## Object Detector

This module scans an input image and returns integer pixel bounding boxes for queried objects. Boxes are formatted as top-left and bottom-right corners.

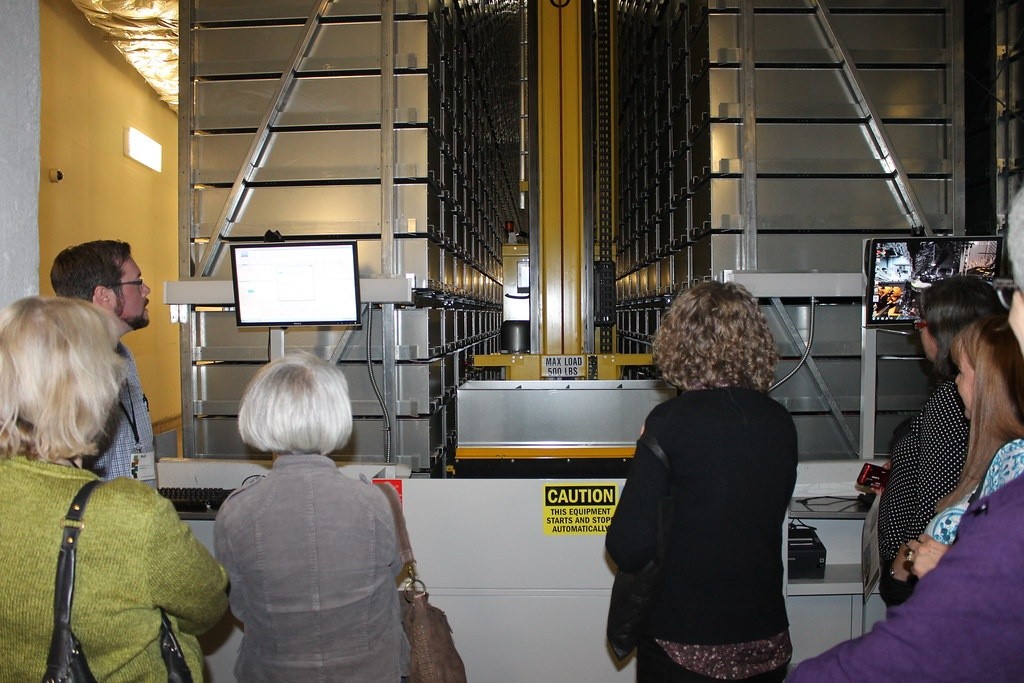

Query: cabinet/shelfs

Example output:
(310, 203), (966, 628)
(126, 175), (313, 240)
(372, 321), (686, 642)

(786, 594), (888, 664)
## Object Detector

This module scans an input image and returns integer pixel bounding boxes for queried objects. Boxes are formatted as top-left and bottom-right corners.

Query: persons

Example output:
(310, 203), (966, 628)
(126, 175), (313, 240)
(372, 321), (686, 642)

(0, 240), (230, 683)
(605, 280), (799, 682)
(212, 353), (412, 683)
(782, 186), (1023, 683)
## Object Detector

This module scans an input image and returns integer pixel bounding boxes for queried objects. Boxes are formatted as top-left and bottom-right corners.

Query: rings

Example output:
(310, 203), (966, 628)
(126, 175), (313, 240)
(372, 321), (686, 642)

(907, 551), (912, 560)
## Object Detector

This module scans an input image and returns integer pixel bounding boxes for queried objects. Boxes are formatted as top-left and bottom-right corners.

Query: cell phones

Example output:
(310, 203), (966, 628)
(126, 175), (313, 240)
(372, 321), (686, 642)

(857, 463), (891, 490)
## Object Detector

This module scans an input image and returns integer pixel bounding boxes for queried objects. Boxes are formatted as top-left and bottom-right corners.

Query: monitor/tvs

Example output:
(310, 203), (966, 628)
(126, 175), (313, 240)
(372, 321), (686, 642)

(866, 234), (1004, 327)
(230, 240), (362, 328)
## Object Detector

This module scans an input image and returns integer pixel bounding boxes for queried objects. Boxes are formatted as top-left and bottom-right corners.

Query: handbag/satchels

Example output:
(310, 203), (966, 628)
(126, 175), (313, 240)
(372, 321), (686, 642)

(41, 480), (194, 683)
(377, 481), (467, 683)
(606, 432), (672, 662)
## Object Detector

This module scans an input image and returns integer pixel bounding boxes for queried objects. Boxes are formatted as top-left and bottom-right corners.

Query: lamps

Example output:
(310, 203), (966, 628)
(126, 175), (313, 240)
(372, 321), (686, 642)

(123, 126), (163, 173)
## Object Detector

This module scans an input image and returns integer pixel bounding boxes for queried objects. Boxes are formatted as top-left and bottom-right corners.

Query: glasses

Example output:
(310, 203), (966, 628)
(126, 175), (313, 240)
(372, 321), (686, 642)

(993, 278), (1024, 309)
(915, 320), (930, 332)
(110, 278), (144, 292)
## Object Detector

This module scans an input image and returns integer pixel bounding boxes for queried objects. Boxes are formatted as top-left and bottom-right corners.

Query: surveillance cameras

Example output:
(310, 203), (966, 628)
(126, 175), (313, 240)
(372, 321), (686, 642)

(49, 168), (64, 182)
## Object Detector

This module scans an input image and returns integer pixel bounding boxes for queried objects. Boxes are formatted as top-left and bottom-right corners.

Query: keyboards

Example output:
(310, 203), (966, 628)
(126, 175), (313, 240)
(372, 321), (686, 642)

(159, 486), (234, 511)
